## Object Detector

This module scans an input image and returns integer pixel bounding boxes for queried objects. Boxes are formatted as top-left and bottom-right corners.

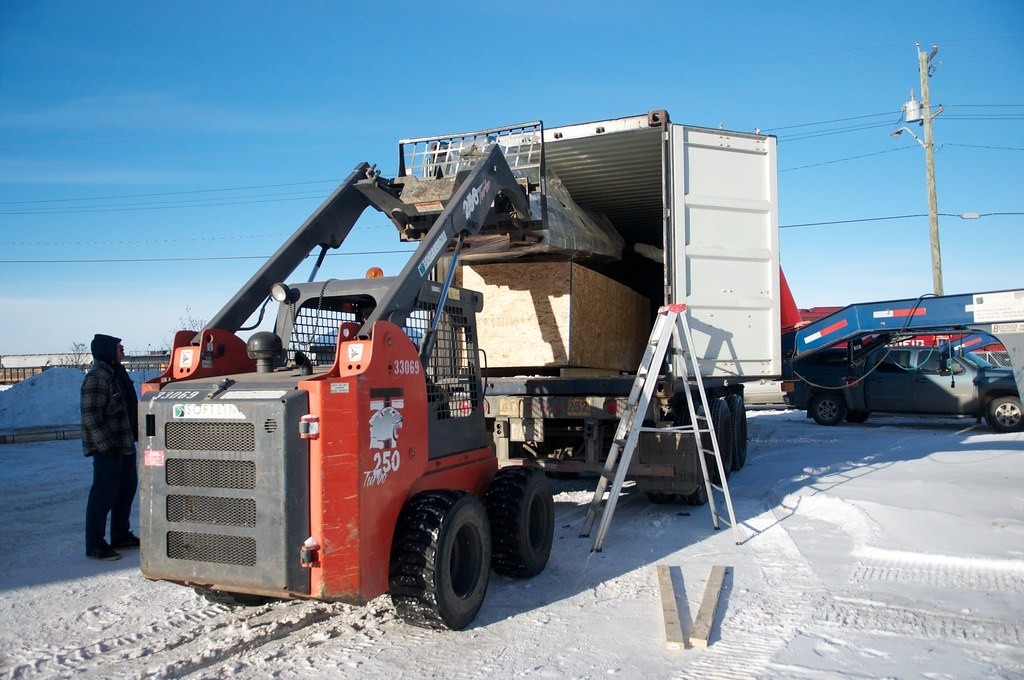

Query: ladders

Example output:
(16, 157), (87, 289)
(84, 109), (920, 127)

(577, 304), (740, 552)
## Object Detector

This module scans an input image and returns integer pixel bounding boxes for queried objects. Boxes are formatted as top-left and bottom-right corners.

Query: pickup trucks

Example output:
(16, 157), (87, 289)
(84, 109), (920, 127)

(780, 346), (1024, 433)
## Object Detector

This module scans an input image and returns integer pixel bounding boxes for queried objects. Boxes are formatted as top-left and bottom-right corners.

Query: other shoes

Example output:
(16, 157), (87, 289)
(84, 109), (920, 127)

(110, 530), (140, 549)
(86, 546), (121, 561)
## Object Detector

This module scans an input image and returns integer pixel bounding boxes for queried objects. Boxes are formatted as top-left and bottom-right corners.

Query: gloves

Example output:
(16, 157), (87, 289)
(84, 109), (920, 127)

(104, 445), (121, 459)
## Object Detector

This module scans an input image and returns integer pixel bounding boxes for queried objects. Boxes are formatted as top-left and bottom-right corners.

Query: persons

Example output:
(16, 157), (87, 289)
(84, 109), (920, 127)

(82, 334), (140, 561)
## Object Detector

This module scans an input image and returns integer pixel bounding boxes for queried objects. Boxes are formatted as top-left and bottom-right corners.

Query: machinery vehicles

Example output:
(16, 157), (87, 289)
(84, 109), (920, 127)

(138, 104), (782, 631)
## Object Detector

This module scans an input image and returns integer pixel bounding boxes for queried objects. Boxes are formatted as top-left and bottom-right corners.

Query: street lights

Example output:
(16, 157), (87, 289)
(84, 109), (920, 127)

(892, 126), (943, 298)
